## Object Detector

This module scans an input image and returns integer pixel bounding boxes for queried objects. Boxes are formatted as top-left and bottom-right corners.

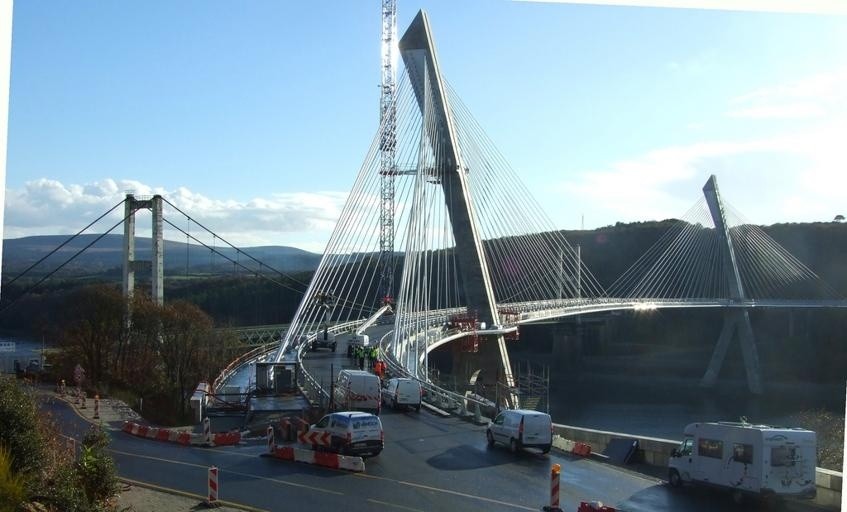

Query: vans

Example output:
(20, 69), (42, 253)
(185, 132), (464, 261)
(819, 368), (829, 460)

(334, 369), (422, 411)
(668, 422), (816, 505)
(486, 409), (553, 454)
(311, 411), (384, 455)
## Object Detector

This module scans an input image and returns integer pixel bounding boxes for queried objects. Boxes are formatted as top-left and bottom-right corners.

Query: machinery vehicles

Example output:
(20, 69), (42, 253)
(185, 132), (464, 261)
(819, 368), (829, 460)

(312, 293), (336, 352)
(348, 304), (393, 346)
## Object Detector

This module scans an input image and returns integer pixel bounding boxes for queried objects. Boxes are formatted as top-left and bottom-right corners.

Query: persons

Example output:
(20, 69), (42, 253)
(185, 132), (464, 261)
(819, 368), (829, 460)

(379, 378), (387, 389)
(348, 342), (386, 377)
